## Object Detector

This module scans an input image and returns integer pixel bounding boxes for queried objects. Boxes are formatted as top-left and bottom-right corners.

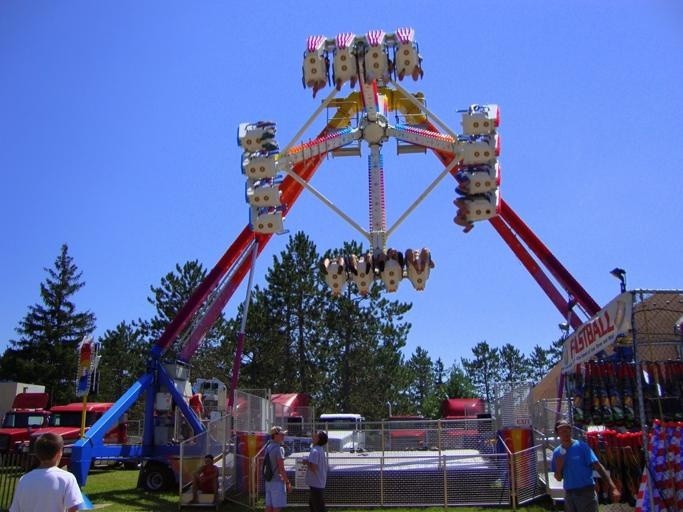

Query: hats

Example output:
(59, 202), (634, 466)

(205, 455), (214, 458)
(555, 420), (571, 429)
(271, 426), (288, 434)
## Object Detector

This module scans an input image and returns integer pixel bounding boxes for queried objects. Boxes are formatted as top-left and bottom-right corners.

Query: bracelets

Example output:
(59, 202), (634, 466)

(285, 480), (290, 485)
(307, 461), (311, 465)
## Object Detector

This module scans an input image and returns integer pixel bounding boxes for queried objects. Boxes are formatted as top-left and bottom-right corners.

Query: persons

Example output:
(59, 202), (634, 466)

(264, 425), (294, 511)
(9, 432), (84, 511)
(189, 392), (208, 444)
(551, 424), (624, 510)
(259, 41), (474, 276)
(300, 431), (327, 510)
(187, 453), (222, 505)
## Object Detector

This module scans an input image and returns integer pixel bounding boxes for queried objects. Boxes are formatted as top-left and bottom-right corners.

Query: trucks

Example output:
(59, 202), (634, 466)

(0, 391), (145, 473)
(269, 392), (492, 453)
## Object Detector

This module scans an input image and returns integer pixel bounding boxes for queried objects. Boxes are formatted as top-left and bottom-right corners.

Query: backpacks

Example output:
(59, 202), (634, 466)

(263, 446), (278, 481)
